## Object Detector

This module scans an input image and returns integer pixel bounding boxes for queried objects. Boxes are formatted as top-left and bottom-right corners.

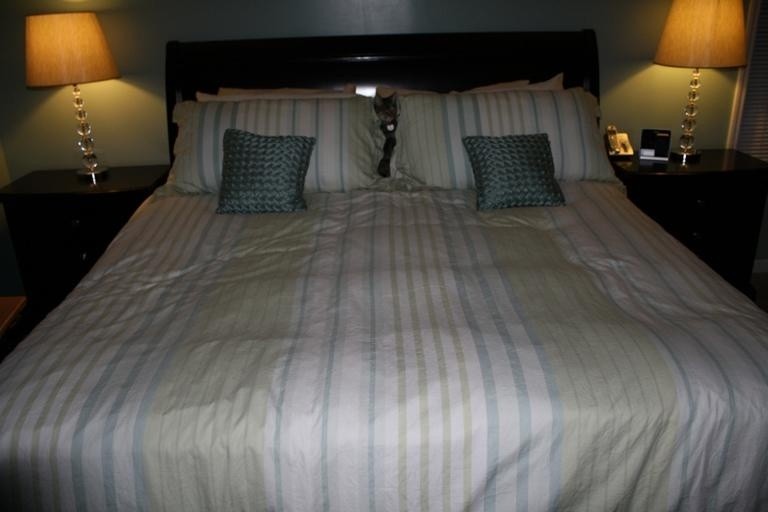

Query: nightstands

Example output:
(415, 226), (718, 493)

(608, 146), (767, 294)
(1, 166), (171, 315)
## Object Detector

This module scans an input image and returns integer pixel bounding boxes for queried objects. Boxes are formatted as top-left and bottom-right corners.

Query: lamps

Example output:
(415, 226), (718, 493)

(654, 2), (751, 164)
(23, 13), (120, 186)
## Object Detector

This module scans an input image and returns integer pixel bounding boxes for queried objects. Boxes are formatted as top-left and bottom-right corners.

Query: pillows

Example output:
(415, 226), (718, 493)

(387, 92), (617, 191)
(173, 96), (386, 196)
(462, 132), (567, 214)
(214, 128), (317, 214)
(500, 74), (568, 92)
(195, 92), (361, 102)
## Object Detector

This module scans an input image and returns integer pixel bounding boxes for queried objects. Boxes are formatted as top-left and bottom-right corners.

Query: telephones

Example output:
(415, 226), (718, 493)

(606, 120), (634, 156)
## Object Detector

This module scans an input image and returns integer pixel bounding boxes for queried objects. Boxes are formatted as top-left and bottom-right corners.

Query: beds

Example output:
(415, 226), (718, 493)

(2, 30), (767, 511)
